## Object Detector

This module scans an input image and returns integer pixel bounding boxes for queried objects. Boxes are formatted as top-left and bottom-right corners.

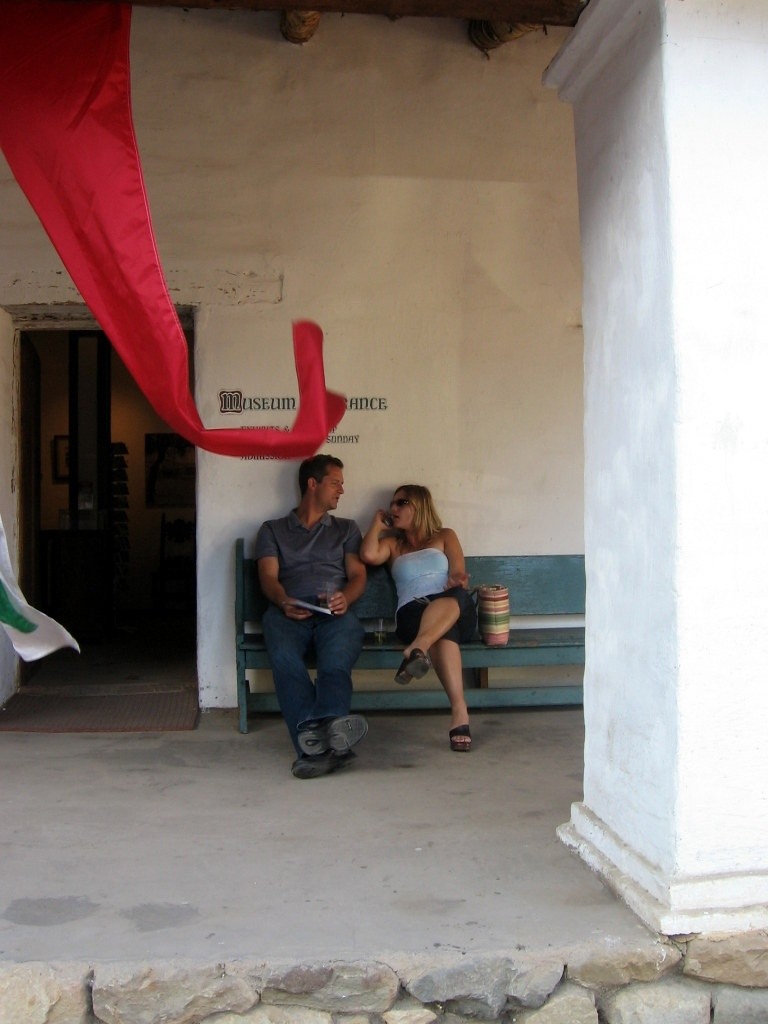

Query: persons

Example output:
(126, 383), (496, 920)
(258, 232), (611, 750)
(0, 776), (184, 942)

(360, 484), (478, 752)
(254, 455), (369, 780)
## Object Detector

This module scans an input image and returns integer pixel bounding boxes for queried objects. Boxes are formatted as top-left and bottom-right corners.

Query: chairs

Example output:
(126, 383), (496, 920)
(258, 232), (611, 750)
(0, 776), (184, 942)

(149, 513), (196, 617)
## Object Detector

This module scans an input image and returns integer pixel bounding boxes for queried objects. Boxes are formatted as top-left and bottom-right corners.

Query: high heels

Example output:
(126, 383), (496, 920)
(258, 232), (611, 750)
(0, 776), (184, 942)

(394, 648), (431, 685)
(449, 724), (472, 750)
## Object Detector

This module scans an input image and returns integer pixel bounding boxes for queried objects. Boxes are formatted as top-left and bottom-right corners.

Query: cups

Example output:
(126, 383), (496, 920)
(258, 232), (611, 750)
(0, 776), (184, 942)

(373, 618), (387, 647)
(320, 582), (337, 609)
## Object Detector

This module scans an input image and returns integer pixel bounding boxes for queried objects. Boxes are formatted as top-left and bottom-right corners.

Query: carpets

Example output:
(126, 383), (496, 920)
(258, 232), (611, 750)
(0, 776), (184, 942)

(0, 688), (200, 733)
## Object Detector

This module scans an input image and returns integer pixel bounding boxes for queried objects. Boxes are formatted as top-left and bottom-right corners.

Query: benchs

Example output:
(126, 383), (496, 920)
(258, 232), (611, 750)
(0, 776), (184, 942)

(233, 538), (586, 736)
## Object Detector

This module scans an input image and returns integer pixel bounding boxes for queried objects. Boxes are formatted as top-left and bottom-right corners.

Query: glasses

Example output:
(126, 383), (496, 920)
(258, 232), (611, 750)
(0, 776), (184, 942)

(390, 499), (411, 507)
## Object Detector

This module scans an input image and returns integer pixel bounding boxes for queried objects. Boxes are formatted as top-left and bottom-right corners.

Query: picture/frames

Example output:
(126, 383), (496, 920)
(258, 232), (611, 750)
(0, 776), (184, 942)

(51, 433), (69, 485)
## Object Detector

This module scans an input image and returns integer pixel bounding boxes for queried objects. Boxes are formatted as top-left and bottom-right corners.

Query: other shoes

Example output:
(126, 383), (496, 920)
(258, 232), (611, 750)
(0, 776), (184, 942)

(297, 713), (367, 757)
(292, 746), (359, 780)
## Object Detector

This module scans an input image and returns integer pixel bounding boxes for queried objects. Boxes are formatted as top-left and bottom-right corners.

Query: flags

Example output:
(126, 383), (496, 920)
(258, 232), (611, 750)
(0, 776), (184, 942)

(0, 515), (82, 662)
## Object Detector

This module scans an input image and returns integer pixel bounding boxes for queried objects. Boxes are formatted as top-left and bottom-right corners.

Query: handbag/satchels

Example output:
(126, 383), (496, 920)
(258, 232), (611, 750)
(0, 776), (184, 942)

(470, 585), (509, 646)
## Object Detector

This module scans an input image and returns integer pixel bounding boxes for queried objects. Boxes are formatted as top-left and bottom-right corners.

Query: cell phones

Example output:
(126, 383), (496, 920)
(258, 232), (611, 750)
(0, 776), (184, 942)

(385, 518), (393, 526)
